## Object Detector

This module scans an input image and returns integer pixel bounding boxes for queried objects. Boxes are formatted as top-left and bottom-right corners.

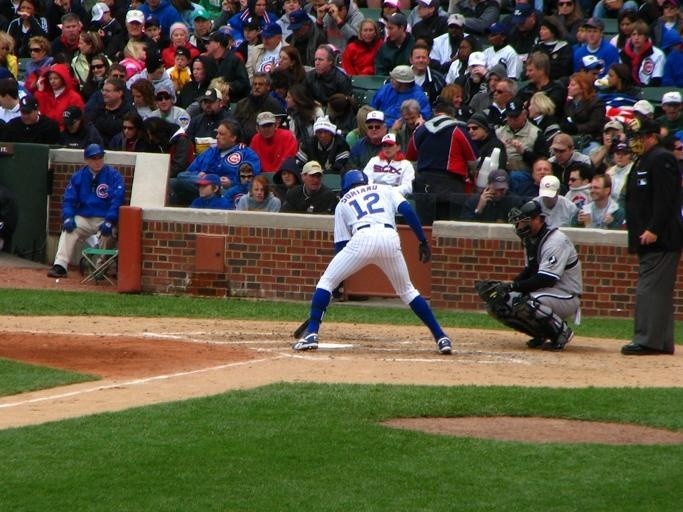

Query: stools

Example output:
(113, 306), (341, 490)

(79, 247), (118, 286)
(77, 239), (101, 277)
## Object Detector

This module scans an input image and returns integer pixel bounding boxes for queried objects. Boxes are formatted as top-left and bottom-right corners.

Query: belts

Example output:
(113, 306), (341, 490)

(357, 222), (396, 231)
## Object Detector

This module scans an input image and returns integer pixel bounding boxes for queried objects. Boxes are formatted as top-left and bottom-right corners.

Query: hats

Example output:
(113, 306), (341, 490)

(258, 21), (282, 38)
(468, 51), (487, 67)
(541, 15), (564, 40)
(504, 98), (525, 118)
(147, 55), (164, 74)
(602, 120), (624, 132)
(384, 0), (401, 9)
(90, 2), (111, 22)
(614, 142), (628, 154)
(219, 26), (236, 43)
(144, 14), (160, 24)
(538, 175), (561, 199)
(193, 8), (210, 23)
(84, 143), (106, 158)
(364, 109), (386, 124)
(391, 65), (416, 84)
(660, 91), (683, 105)
(446, 12), (466, 27)
(313, 115), (338, 135)
(416, 0), (439, 10)
(549, 133), (574, 151)
(487, 169), (510, 190)
(242, 13), (262, 30)
(62, 105), (83, 126)
(379, 132), (403, 146)
(575, 55), (605, 71)
(192, 173), (221, 186)
(511, 3), (533, 24)
(631, 100), (655, 115)
(467, 109), (490, 132)
(201, 30), (230, 49)
(199, 88), (223, 103)
(485, 22), (507, 35)
(286, 10), (310, 29)
(256, 111), (276, 126)
(174, 46), (191, 62)
(301, 160), (324, 176)
(583, 18), (606, 32)
(154, 85), (172, 97)
(384, 12), (407, 31)
(169, 22), (190, 43)
(125, 9), (145, 24)
(15, 95), (39, 114)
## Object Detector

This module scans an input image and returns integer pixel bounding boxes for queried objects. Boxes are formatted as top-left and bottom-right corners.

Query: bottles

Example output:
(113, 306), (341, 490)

(306, 205), (315, 213)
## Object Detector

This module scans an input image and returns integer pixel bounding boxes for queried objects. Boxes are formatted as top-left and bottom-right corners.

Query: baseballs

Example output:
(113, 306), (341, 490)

(55, 278), (60, 283)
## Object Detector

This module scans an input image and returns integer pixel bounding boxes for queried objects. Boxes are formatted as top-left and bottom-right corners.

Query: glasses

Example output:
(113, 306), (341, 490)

(157, 95), (170, 102)
(559, 1), (574, 6)
(113, 74), (125, 79)
(570, 177), (582, 183)
(240, 174), (253, 179)
(30, 48), (41, 53)
(123, 125), (134, 130)
(91, 64), (104, 69)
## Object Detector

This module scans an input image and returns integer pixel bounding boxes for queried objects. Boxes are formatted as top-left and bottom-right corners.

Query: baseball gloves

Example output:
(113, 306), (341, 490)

(477, 280), (516, 303)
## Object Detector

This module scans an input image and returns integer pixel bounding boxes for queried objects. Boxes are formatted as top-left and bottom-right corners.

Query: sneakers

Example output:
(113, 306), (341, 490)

(291, 329), (320, 351)
(437, 336), (453, 354)
(543, 324), (575, 352)
(621, 337), (675, 357)
(527, 318), (555, 349)
(47, 264), (68, 279)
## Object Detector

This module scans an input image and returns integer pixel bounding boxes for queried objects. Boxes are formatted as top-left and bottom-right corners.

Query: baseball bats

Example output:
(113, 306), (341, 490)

(293, 287), (344, 339)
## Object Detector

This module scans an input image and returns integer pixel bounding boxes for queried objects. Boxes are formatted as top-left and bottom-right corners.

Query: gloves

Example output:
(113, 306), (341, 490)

(97, 220), (114, 238)
(62, 217), (78, 234)
(417, 240), (431, 264)
(331, 280), (344, 299)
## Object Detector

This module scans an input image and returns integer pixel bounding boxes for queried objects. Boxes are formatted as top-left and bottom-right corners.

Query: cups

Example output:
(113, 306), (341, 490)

(476, 147), (500, 187)
(583, 204), (593, 226)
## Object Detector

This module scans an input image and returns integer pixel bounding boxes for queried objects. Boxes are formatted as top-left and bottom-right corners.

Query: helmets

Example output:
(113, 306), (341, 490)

(507, 200), (543, 239)
(340, 169), (368, 193)
